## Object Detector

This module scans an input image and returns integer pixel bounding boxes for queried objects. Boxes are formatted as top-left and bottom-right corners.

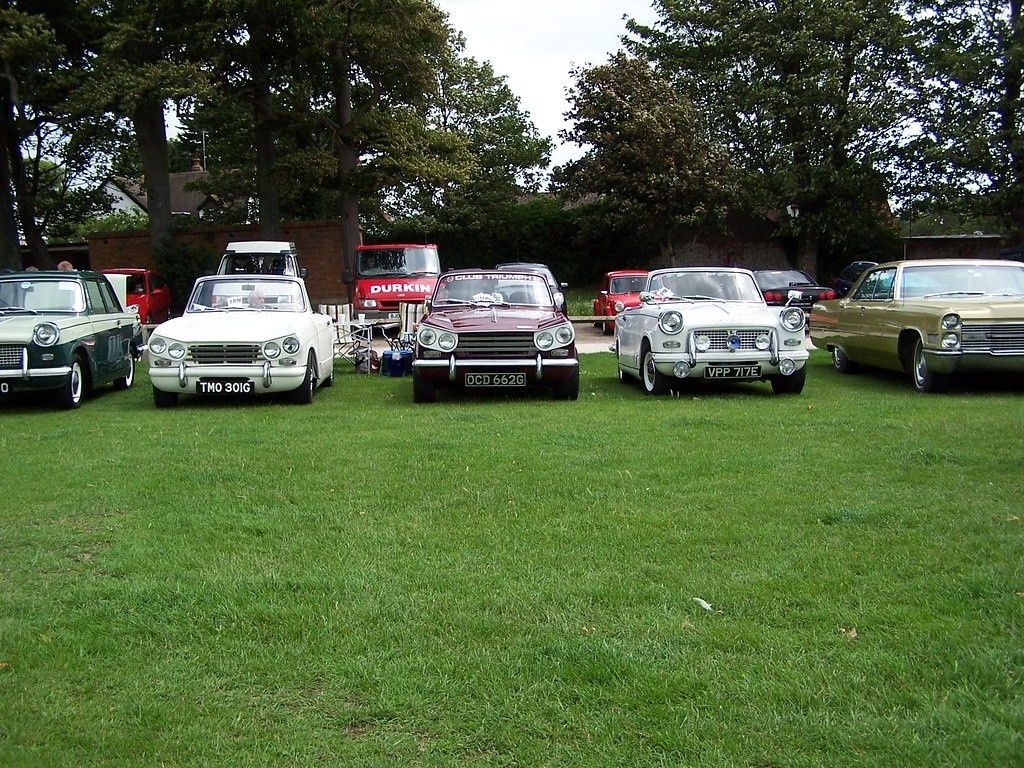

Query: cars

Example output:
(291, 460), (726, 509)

(809, 258), (1024, 392)
(148, 275), (334, 408)
(412, 268), (580, 403)
(593, 271), (670, 336)
(753, 270), (836, 328)
(613, 266), (809, 396)
(487, 263), (568, 320)
(100, 268), (171, 332)
(0, 269), (145, 409)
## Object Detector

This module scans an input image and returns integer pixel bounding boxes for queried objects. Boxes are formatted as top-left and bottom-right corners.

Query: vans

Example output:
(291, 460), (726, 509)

(211, 241), (307, 310)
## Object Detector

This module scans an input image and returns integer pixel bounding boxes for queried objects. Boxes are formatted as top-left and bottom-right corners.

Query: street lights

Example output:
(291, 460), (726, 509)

(201, 127), (209, 171)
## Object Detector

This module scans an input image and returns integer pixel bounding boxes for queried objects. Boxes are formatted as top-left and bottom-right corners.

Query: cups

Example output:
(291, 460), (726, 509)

(393, 353), (402, 360)
(338, 314), (345, 324)
(358, 314), (365, 322)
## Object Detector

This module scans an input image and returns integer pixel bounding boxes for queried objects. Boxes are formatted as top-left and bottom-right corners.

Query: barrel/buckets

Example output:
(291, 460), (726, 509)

(390, 358), (403, 377)
(383, 350), (395, 375)
(398, 350), (413, 373)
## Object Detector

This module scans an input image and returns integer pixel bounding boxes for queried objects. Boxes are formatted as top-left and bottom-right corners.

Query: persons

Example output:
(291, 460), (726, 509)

(58, 261), (78, 271)
(364, 257), (386, 276)
(133, 284), (143, 293)
(247, 290), (272, 309)
(26, 267), (38, 272)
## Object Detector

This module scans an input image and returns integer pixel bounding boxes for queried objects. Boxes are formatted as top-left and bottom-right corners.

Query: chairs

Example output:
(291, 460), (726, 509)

(508, 292), (535, 304)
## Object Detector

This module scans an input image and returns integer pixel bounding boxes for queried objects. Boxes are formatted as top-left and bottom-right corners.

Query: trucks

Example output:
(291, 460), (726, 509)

(342, 243), (455, 340)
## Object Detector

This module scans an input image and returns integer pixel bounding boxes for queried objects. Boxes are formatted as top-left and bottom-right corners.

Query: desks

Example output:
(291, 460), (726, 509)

(333, 318), (401, 375)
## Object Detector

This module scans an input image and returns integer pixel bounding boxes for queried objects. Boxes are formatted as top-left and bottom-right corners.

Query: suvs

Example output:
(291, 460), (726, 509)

(830, 260), (922, 302)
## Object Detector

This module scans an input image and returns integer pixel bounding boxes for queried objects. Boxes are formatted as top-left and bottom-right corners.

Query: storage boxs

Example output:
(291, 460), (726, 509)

(383, 351), (414, 376)
